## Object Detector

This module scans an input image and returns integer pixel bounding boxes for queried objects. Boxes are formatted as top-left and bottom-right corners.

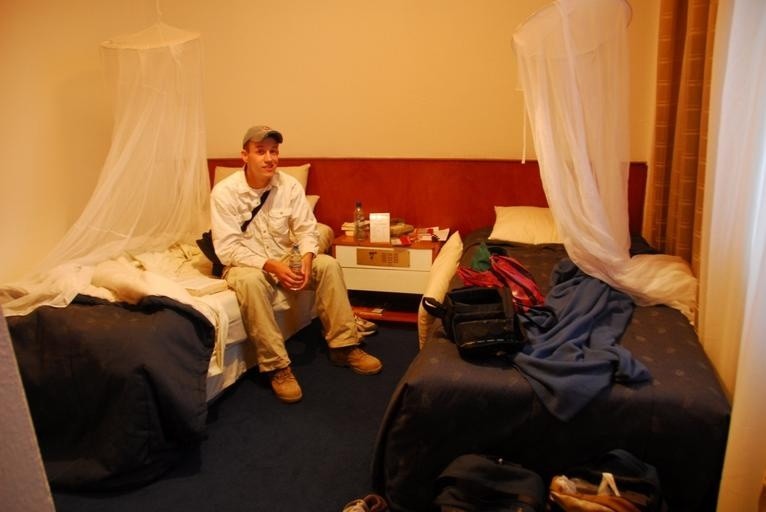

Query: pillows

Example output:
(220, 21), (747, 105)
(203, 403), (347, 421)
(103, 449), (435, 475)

(215, 164), (310, 201)
(304, 197), (320, 208)
(317, 222), (332, 248)
(489, 204), (565, 246)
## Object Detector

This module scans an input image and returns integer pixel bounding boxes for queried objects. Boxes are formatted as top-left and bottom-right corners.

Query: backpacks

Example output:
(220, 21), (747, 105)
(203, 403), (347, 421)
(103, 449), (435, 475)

(441, 255), (547, 361)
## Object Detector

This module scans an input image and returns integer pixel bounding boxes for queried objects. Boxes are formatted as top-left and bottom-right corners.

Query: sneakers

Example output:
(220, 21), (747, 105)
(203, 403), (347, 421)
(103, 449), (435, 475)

(329, 345), (384, 375)
(342, 494), (390, 512)
(321, 312), (378, 340)
(268, 365), (303, 403)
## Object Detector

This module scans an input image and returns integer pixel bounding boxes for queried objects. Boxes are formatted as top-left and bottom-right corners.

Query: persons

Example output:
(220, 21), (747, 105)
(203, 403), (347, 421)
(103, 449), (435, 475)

(209, 126), (383, 404)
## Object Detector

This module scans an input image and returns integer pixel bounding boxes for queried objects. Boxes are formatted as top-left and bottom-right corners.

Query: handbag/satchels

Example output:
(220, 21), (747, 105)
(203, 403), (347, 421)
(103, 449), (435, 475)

(196, 228), (224, 276)
(549, 449), (670, 511)
(432, 454), (547, 511)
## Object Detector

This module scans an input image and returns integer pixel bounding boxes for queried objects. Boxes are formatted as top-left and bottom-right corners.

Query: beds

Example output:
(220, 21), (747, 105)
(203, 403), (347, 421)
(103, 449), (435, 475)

(1, 222), (327, 499)
(376, 204), (731, 510)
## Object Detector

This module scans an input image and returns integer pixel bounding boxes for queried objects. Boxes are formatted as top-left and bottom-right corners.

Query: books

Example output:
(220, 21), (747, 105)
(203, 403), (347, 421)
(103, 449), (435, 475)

(341, 222), (369, 236)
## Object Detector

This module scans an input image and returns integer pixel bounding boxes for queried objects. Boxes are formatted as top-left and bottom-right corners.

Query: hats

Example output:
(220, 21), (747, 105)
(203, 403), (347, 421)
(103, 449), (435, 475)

(242, 126), (283, 149)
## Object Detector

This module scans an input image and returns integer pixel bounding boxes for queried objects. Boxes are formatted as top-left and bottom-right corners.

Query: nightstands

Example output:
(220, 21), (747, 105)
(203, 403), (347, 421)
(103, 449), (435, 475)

(329, 231), (439, 332)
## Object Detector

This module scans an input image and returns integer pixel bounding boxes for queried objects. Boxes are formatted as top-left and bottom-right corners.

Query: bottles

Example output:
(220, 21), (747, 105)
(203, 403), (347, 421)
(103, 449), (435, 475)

(353, 202), (365, 241)
(289, 244), (302, 295)
